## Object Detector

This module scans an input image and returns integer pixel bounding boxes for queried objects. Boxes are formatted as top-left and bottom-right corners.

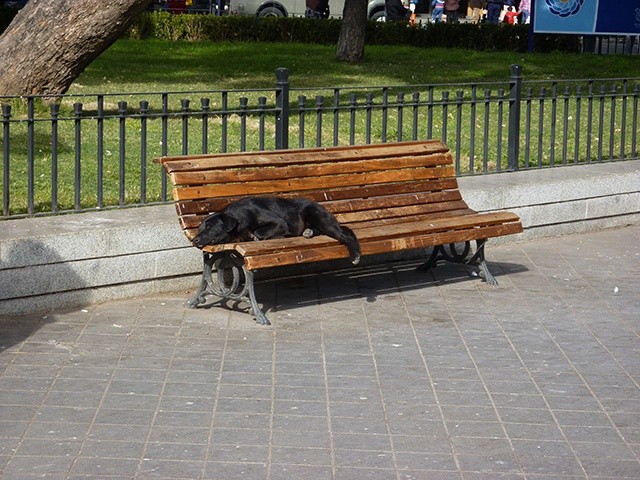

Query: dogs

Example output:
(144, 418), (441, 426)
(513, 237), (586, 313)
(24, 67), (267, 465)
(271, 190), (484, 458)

(191, 196), (361, 266)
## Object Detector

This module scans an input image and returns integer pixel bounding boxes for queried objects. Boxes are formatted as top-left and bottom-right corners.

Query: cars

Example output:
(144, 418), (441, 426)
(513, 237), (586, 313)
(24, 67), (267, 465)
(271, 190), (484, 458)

(213, 0), (392, 25)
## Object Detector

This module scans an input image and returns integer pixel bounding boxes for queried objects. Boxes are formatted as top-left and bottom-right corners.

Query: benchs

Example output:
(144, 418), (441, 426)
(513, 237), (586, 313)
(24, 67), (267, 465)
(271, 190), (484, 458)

(154, 139), (523, 324)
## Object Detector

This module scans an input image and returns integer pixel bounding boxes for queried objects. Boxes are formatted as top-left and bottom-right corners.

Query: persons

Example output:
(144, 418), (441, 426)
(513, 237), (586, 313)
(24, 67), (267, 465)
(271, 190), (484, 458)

(504, 4), (520, 28)
(406, 0), (417, 26)
(429, 0), (442, 25)
(446, 0), (461, 25)
(519, 0), (532, 27)
